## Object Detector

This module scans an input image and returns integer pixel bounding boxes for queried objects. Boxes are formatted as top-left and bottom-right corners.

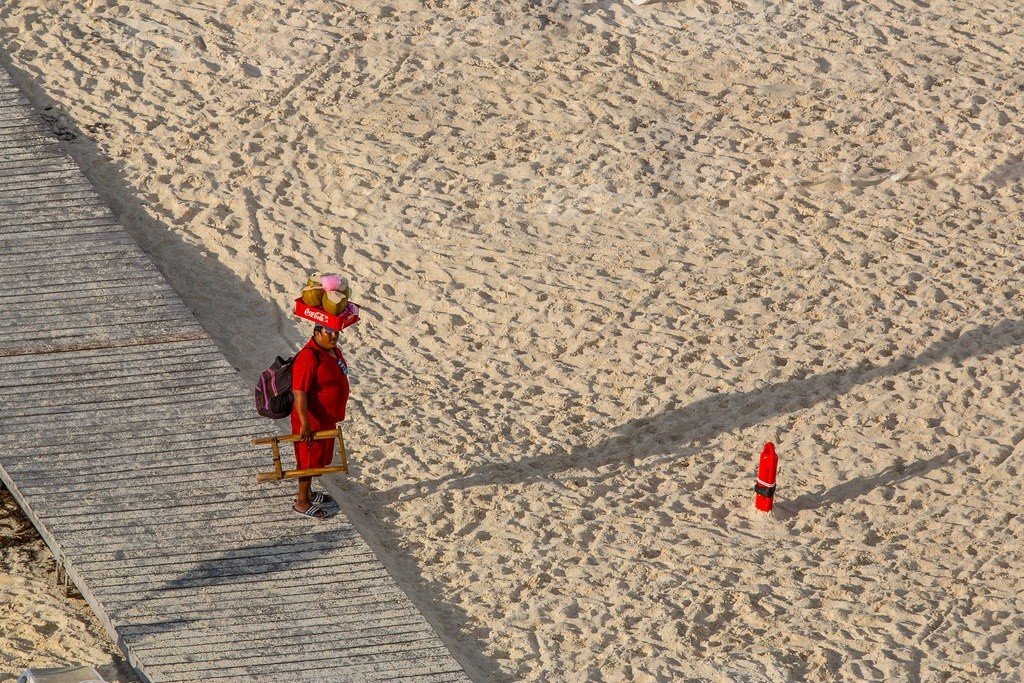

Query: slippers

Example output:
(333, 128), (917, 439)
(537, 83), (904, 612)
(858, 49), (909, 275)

(294, 491), (331, 504)
(292, 504), (329, 519)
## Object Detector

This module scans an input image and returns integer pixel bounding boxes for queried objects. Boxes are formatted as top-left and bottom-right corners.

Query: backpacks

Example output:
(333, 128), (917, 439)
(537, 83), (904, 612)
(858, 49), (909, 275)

(254, 348), (320, 419)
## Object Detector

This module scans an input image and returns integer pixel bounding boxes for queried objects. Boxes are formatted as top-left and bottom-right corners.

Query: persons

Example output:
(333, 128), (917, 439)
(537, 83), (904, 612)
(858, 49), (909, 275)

(291, 322), (349, 519)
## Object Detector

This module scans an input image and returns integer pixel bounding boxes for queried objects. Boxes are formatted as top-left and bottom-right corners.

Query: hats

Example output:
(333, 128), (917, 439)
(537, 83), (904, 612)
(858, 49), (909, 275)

(315, 322), (344, 333)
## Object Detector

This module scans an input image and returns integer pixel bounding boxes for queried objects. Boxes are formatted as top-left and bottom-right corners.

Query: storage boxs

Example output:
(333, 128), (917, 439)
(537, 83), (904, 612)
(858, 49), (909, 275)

(292, 294), (361, 331)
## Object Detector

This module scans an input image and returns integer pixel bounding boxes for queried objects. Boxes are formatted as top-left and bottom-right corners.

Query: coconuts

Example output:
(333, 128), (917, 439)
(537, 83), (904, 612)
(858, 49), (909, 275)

(301, 272), (350, 316)
